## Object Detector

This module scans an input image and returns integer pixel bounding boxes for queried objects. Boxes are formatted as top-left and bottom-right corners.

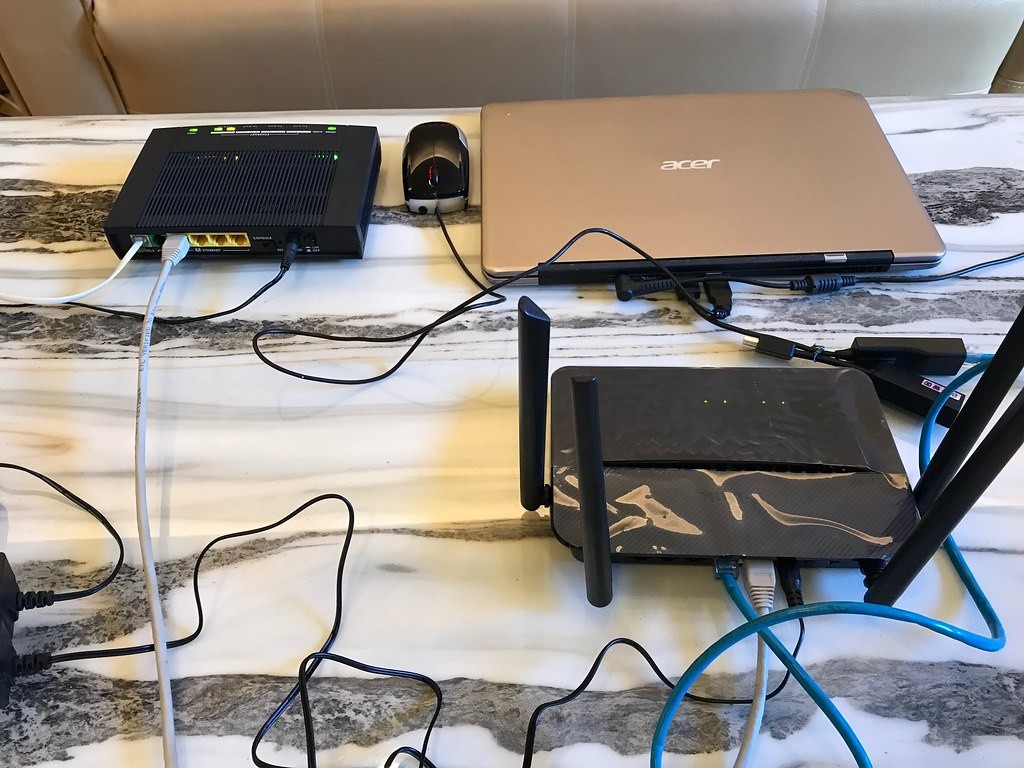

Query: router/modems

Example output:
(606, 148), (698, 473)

(103, 124), (383, 262)
(513, 294), (1024, 606)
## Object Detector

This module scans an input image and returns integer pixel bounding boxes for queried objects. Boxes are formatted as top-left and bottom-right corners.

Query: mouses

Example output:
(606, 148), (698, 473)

(402, 121), (469, 215)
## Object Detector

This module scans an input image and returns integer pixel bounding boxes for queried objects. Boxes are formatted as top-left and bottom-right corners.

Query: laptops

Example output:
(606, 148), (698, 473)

(479, 88), (944, 284)
